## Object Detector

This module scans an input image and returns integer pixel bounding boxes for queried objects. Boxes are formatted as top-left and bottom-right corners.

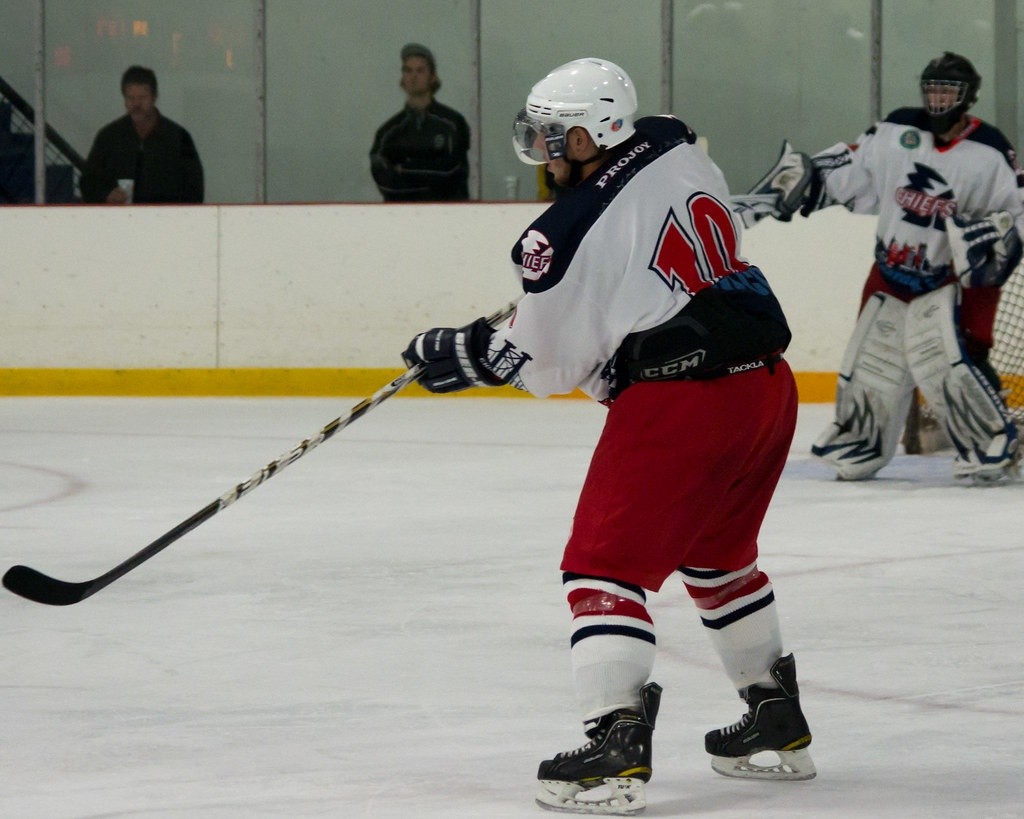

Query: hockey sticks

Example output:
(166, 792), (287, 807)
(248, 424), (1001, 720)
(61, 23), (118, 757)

(2, 295), (524, 605)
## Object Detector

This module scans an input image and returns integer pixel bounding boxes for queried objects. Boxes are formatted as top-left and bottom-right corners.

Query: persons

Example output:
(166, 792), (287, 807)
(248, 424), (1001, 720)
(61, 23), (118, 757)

(78, 64), (205, 209)
(408, 57), (810, 786)
(756, 54), (1020, 483)
(368, 42), (472, 205)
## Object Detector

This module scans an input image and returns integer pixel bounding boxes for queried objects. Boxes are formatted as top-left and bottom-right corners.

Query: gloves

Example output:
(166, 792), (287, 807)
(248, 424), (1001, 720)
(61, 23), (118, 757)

(401, 316), (506, 393)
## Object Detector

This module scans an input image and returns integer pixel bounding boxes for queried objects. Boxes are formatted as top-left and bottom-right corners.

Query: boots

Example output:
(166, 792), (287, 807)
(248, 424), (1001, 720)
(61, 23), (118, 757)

(536, 681), (664, 815)
(706, 653), (817, 781)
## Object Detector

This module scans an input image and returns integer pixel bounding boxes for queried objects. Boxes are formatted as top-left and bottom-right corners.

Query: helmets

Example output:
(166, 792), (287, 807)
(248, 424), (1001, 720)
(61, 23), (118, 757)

(919, 52), (980, 135)
(512, 58), (640, 167)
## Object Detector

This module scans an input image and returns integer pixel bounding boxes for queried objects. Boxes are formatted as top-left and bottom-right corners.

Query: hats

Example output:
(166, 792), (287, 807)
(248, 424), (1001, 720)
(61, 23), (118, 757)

(402, 43), (433, 65)
